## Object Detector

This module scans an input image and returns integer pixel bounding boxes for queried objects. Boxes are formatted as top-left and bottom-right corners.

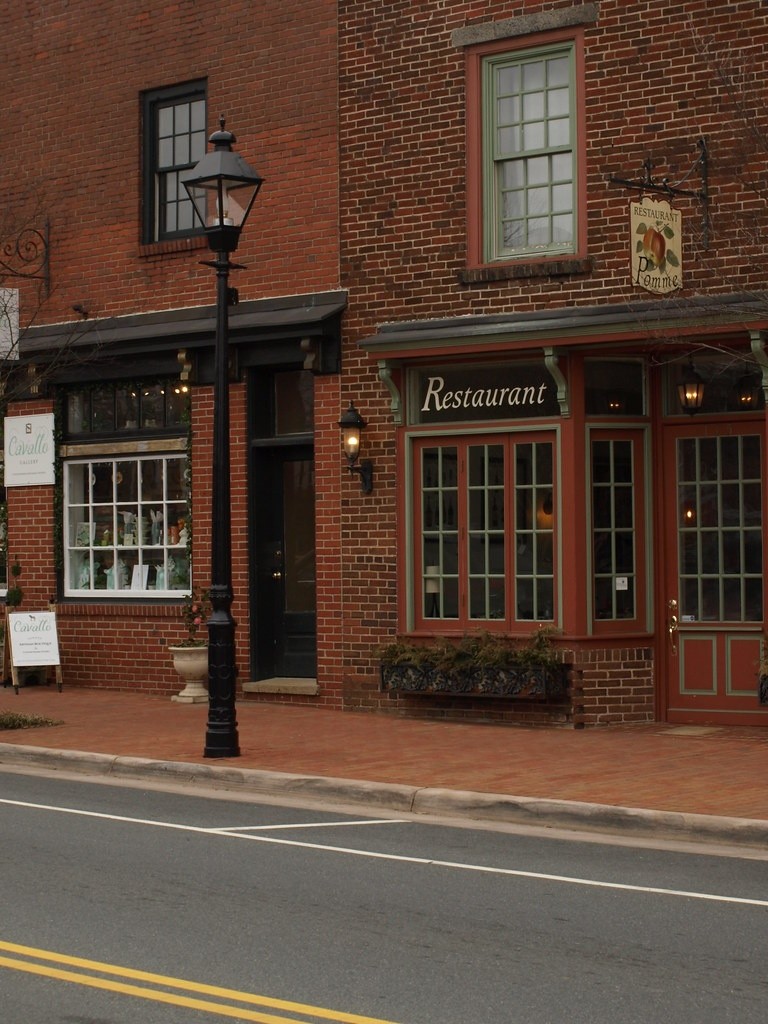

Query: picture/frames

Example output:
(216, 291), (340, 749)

(75, 521), (96, 547)
(480, 456), (528, 547)
(422, 451), (459, 543)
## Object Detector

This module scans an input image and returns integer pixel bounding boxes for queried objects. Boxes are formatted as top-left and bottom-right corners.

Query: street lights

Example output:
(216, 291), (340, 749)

(177, 114), (267, 755)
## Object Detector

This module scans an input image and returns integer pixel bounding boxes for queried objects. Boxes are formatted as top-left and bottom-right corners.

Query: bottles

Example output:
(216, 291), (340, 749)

(159, 529), (163, 545)
(151, 522), (161, 545)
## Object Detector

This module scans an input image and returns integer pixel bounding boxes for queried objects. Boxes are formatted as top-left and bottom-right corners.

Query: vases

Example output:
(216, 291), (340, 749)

(168, 645), (208, 704)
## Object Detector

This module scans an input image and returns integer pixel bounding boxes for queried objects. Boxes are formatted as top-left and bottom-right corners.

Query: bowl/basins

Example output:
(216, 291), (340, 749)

(131, 516), (150, 545)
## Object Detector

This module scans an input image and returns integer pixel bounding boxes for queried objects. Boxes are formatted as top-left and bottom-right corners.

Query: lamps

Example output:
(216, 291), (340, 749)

(543, 491), (553, 515)
(72, 305), (88, 315)
(335, 398), (374, 494)
(733, 362), (761, 411)
(676, 347), (707, 416)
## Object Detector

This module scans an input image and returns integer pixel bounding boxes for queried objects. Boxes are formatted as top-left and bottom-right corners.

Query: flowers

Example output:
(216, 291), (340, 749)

(176, 592), (214, 648)
